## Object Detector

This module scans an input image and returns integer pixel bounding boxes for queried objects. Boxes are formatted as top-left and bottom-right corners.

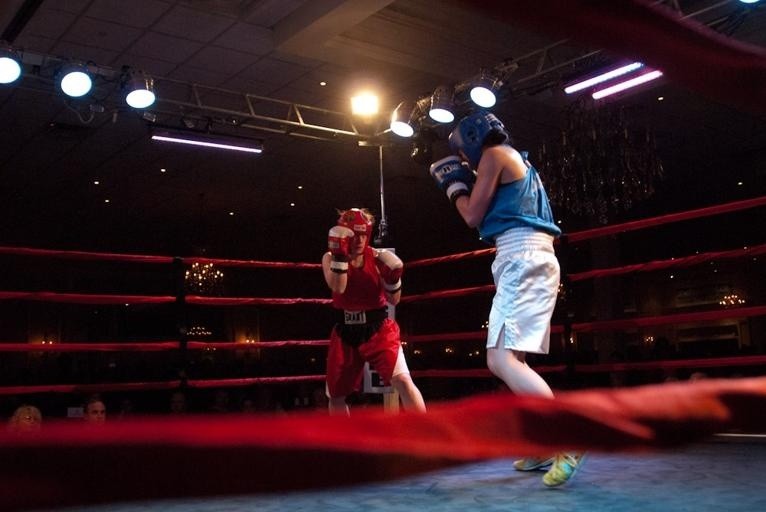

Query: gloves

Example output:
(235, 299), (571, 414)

(376, 250), (403, 292)
(430, 156), (478, 199)
(330, 225), (354, 274)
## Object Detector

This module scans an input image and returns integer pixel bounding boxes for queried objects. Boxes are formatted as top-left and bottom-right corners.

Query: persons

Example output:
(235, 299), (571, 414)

(321, 204), (428, 417)
(7, 340), (766, 431)
(428, 110), (590, 490)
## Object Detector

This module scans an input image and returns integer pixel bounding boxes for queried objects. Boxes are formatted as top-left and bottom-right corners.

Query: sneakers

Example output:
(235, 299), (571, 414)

(513, 450), (590, 487)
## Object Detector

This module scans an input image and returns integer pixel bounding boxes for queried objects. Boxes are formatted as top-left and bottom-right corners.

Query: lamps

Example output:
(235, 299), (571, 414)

(144, 121), (266, 158)
(0, 44), (159, 114)
(387, 56), (520, 139)
(557, 58), (661, 104)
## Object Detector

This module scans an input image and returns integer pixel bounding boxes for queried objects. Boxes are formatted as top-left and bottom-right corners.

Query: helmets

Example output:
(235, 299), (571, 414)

(446, 107), (507, 169)
(338, 206), (374, 257)
